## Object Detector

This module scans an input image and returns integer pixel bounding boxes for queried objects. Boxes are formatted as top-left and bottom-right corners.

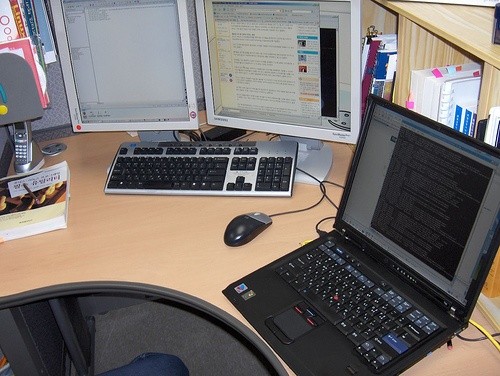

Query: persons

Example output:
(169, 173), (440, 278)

(362, 32), (397, 113)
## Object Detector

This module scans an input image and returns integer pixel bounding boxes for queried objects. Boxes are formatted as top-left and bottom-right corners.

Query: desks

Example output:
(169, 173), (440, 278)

(0, 111), (500, 376)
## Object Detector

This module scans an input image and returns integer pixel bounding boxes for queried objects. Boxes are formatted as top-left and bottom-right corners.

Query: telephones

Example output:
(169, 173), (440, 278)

(13, 120), (45, 174)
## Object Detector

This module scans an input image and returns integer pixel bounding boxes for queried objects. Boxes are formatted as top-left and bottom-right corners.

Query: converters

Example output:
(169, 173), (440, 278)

(201, 126), (246, 141)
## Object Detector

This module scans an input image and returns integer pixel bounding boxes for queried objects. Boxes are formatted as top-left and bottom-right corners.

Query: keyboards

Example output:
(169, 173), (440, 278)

(104, 142), (298, 197)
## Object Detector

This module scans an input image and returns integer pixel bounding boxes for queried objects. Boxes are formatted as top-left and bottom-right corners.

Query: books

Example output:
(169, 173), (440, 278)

(407, 62), (483, 140)
(0, 0), (50, 110)
(477, 107), (500, 148)
(0, 160), (71, 243)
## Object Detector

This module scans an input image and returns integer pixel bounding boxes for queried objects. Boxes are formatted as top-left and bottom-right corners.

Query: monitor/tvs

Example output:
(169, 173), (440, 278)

(50, 0), (199, 143)
(194, 0), (362, 185)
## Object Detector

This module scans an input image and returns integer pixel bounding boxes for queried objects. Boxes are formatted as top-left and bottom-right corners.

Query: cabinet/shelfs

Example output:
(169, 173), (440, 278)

(360, 0), (500, 334)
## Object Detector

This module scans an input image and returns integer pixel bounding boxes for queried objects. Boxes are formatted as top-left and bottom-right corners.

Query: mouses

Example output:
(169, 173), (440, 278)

(223, 212), (272, 247)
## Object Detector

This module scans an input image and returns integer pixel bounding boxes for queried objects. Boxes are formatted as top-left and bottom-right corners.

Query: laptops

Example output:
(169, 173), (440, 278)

(222, 93), (500, 376)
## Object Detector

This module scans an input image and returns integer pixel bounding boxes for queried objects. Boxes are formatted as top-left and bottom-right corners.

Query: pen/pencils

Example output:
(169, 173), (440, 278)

(24, 183), (41, 205)
